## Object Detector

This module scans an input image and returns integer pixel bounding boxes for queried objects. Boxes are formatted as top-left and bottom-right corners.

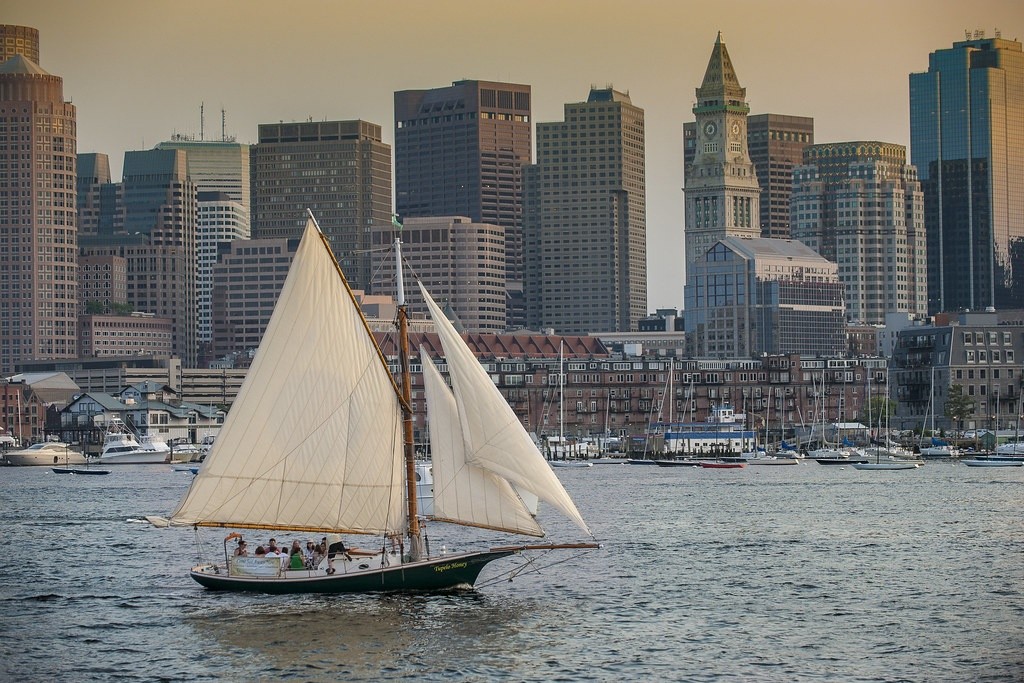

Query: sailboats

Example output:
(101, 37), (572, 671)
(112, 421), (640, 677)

(535, 340), (593, 467)
(587, 357), (1024, 471)
(126, 208), (604, 595)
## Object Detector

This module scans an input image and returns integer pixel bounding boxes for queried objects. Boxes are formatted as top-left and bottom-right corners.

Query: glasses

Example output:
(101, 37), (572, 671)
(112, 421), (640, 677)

(242, 544), (247, 546)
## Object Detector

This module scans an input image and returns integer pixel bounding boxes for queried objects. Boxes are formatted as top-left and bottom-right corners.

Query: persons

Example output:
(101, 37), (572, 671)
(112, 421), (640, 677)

(290, 537), (326, 570)
(264, 538), (280, 555)
(278, 547), (290, 568)
(264, 546), (278, 557)
(326, 534), (352, 574)
(255, 546), (265, 557)
(234, 540), (247, 556)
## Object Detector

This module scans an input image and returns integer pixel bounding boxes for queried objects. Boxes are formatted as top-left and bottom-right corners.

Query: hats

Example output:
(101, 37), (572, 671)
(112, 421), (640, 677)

(306, 539), (314, 543)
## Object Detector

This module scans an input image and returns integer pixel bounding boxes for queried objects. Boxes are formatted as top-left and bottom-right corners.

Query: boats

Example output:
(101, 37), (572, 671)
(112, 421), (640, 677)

(404, 459), (434, 487)
(199, 436), (216, 462)
(3, 434), (101, 467)
(89, 412), (170, 464)
(165, 437), (201, 463)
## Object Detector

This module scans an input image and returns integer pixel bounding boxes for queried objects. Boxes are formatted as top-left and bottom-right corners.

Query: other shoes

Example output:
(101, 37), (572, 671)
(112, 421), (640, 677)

(345, 554), (352, 561)
(328, 572), (334, 575)
